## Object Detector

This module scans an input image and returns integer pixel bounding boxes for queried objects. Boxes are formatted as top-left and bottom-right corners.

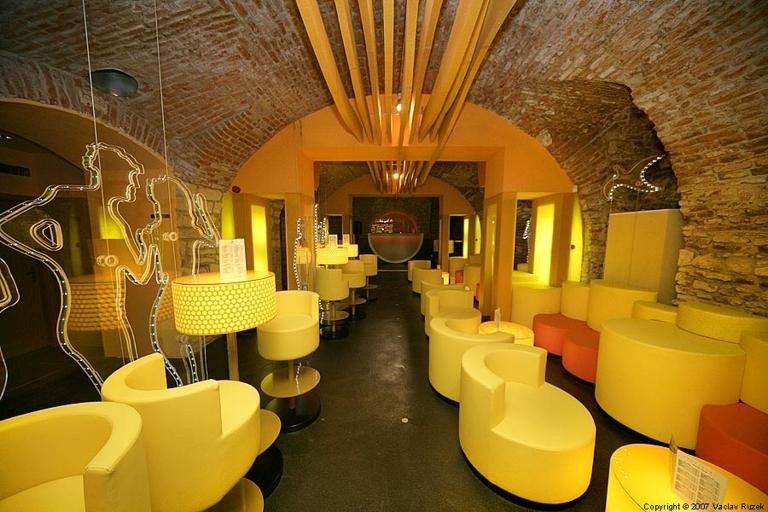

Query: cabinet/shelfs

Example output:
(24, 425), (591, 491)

(604, 208), (686, 306)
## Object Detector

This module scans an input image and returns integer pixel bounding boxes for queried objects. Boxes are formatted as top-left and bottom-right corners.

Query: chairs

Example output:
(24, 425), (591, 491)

(1, 402), (149, 511)
(101, 351), (263, 512)
(257, 290), (321, 433)
(316, 268), (349, 339)
(334, 260), (366, 320)
(359, 254), (377, 302)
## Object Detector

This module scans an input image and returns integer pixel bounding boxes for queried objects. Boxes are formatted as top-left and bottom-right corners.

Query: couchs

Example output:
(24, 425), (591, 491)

(428, 318), (514, 403)
(420, 280), (473, 316)
(691, 331), (768, 495)
(425, 288), (481, 336)
(532, 281), (657, 383)
(412, 267), (444, 294)
(460, 342), (597, 506)
(408, 260), (431, 282)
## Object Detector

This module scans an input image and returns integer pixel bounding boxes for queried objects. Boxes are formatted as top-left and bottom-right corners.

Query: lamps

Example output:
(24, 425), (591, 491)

(396, 98), (402, 113)
